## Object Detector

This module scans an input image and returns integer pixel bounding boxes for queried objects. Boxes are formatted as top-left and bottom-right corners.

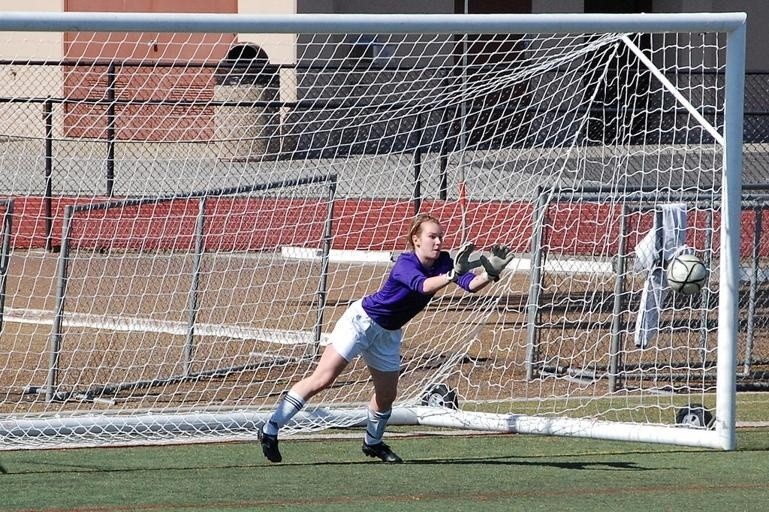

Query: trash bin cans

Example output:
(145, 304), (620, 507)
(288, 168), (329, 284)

(210, 42), (281, 163)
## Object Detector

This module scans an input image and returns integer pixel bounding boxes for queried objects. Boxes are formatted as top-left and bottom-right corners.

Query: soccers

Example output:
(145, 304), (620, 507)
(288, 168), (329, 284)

(666, 254), (706, 295)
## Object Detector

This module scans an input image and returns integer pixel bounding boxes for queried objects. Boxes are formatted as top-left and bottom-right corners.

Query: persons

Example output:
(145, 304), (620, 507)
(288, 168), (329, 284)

(257, 215), (515, 463)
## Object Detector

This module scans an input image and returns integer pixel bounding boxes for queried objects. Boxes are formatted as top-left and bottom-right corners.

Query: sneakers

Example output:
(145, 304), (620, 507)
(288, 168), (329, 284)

(362, 438), (402, 463)
(258, 422), (282, 462)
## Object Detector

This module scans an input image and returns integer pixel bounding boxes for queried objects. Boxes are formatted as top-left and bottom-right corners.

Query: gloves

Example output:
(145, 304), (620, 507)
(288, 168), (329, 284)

(480, 243), (515, 280)
(446, 242), (481, 282)
